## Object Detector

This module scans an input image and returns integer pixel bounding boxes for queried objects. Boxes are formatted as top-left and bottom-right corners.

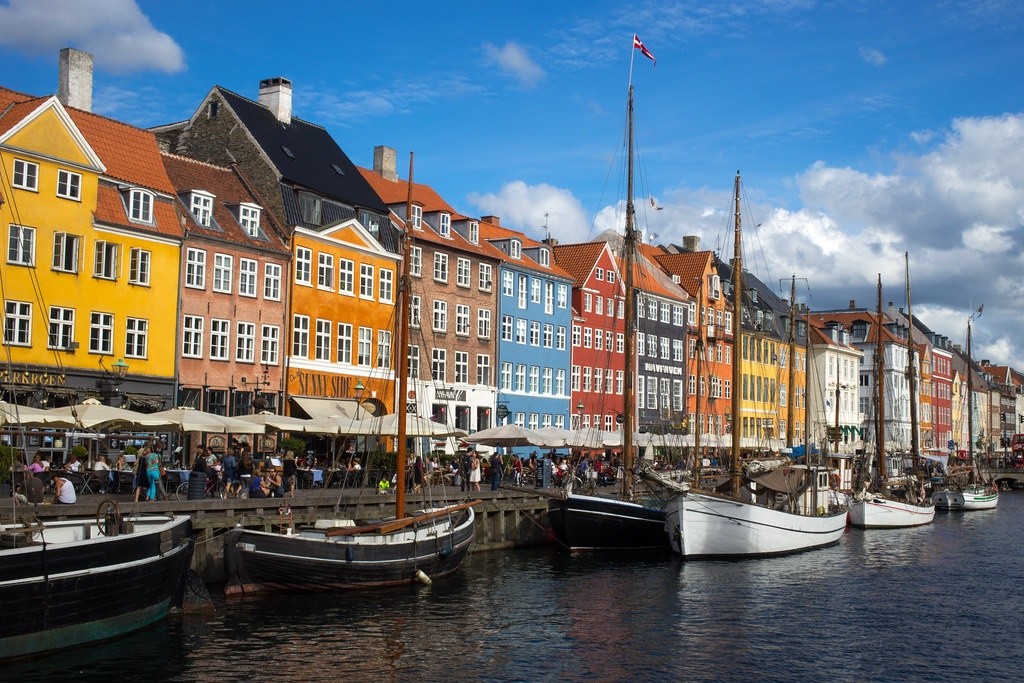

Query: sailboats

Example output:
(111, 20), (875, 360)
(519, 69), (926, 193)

(222, 149), (483, 598)
(494, 33), (1001, 558)
(0, 147), (196, 666)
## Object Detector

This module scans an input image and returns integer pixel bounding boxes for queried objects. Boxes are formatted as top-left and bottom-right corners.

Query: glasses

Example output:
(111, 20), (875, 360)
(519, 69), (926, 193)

(269, 471), (274, 473)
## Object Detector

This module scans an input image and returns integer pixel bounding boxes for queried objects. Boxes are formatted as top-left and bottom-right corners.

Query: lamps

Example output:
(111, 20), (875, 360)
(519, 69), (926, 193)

(99, 359), (129, 383)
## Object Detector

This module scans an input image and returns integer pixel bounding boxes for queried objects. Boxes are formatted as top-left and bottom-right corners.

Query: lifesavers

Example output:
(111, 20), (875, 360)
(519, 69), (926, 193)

(829, 474), (840, 487)
(993, 483), (998, 491)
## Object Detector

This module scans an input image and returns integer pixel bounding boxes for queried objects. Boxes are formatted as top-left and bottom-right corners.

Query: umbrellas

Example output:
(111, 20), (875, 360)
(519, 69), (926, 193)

(458, 423), (564, 456)
(0, 399), (80, 433)
(363, 412), (469, 454)
(574, 427), (638, 456)
(312, 414), (398, 468)
(47, 397), (179, 460)
(532, 425), (603, 454)
(231, 410), (338, 459)
(145, 404), (266, 468)
(613, 428), (785, 456)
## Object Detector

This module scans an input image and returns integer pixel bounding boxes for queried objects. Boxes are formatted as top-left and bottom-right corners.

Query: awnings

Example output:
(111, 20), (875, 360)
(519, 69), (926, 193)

(292, 396), (375, 420)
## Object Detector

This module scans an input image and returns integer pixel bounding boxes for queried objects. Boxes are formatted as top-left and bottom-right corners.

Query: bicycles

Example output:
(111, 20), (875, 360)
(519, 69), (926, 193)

(176, 463), (228, 502)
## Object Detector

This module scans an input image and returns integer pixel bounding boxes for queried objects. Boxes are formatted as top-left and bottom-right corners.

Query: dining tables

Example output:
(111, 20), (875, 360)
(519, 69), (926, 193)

(67, 469), (94, 494)
(166, 470), (190, 491)
(297, 468), (322, 488)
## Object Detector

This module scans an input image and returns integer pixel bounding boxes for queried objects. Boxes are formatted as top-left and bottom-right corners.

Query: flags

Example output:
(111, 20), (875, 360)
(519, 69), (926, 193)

(634, 34), (656, 67)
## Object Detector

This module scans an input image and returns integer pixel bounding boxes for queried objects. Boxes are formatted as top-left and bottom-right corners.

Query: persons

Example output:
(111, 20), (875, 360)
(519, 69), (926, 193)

(430, 447), (490, 492)
(133, 440), (171, 503)
(350, 458), (362, 471)
(10, 450), (83, 493)
(51, 471), (76, 504)
(186, 442), (298, 501)
(93, 455), (131, 493)
(491, 452), (503, 492)
(377, 452), (434, 496)
(14, 469), (46, 507)
(509, 448), (622, 488)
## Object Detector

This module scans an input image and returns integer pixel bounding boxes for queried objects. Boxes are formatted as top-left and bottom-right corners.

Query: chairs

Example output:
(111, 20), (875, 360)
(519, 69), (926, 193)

(10, 470), (51, 495)
(328, 471), (364, 488)
(92, 471), (132, 493)
